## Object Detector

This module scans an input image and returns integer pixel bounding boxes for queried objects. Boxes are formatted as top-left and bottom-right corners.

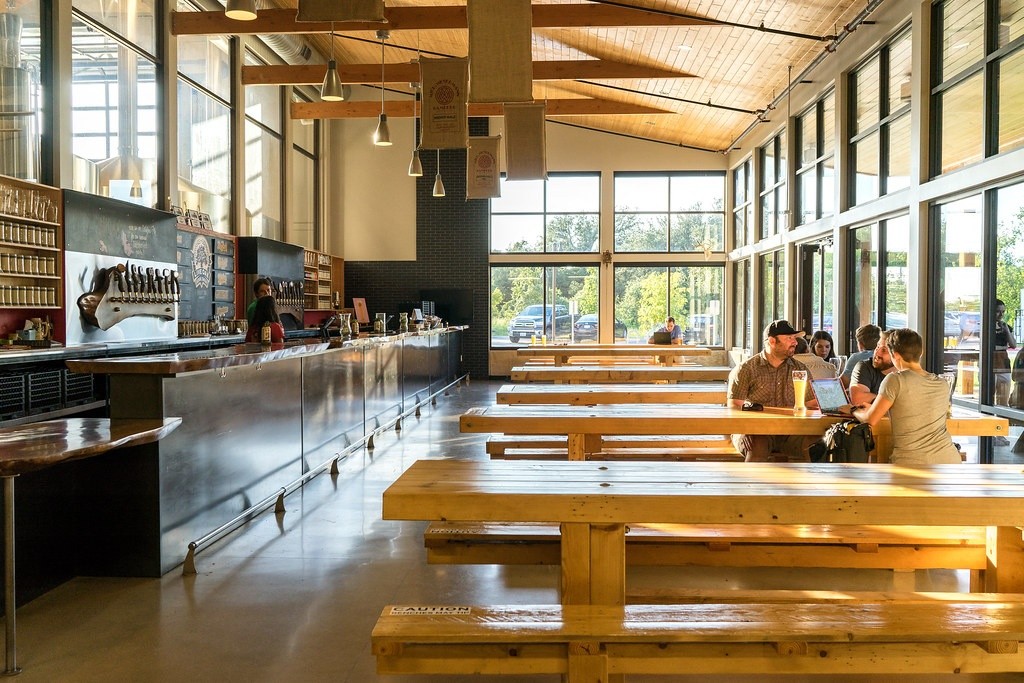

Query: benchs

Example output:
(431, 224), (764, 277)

(371, 435), (1023, 683)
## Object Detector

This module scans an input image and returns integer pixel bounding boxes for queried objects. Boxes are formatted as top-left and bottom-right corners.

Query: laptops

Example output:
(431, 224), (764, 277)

(654, 331), (671, 346)
(810, 378), (856, 419)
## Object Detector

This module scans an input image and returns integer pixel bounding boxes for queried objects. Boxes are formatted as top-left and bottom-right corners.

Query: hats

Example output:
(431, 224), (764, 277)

(764, 319), (806, 337)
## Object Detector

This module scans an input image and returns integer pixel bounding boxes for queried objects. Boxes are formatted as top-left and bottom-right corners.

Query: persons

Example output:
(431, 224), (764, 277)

(988, 299), (1017, 446)
(837, 328), (964, 464)
(793, 322), (899, 462)
(245, 296), (284, 343)
(648, 317), (684, 363)
(727, 319), (819, 463)
(246, 279), (272, 319)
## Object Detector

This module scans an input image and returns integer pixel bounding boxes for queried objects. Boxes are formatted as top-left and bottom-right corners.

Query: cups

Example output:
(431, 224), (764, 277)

(542, 335), (546, 346)
(531, 335), (536, 344)
(678, 334), (683, 345)
(828, 355), (847, 376)
(0, 183), (58, 223)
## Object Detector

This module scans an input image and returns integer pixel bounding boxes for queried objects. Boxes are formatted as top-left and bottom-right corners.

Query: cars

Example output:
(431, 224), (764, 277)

(813, 314), (832, 334)
(884, 312), (981, 336)
(572, 314), (628, 343)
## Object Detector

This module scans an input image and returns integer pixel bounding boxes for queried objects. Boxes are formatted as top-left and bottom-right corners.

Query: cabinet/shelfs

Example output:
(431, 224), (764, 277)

(302, 250), (345, 310)
(0, 172), (64, 310)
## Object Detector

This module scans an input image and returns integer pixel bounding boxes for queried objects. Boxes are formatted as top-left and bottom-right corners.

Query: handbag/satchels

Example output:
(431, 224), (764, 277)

(827, 419), (875, 463)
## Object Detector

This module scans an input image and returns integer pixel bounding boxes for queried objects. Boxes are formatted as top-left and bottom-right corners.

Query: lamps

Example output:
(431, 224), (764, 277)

(407, 82), (424, 177)
(224, 0), (258, 21)
(320, 22), (344, 102)
(433, 149), (446, 197)
(371, 29), (392, 146)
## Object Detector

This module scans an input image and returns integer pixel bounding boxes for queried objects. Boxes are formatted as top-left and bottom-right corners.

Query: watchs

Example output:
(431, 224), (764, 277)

(850, 406), (857, 415)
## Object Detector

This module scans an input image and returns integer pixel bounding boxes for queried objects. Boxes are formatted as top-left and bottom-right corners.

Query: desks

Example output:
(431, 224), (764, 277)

(379, 347), (1023, 606)
(0, 416), (184, 675)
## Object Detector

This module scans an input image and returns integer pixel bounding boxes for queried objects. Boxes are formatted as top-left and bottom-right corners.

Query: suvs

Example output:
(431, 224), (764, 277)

(687, 314), (722, 345)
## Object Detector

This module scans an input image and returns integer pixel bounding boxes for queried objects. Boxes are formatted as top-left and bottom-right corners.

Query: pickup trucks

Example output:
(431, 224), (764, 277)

(509, 304), (580, 343)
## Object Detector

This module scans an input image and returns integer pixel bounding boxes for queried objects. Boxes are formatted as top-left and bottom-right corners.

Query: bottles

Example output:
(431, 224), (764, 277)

(399, 312), (408, 331)
(351, 319), (361, 336)
(339, 312), (352, 336)
(260, 321), (271, 345)
(0, 221), (58, 307)
(791, 370), (807, 411)
(374, 318), (380, 333)
